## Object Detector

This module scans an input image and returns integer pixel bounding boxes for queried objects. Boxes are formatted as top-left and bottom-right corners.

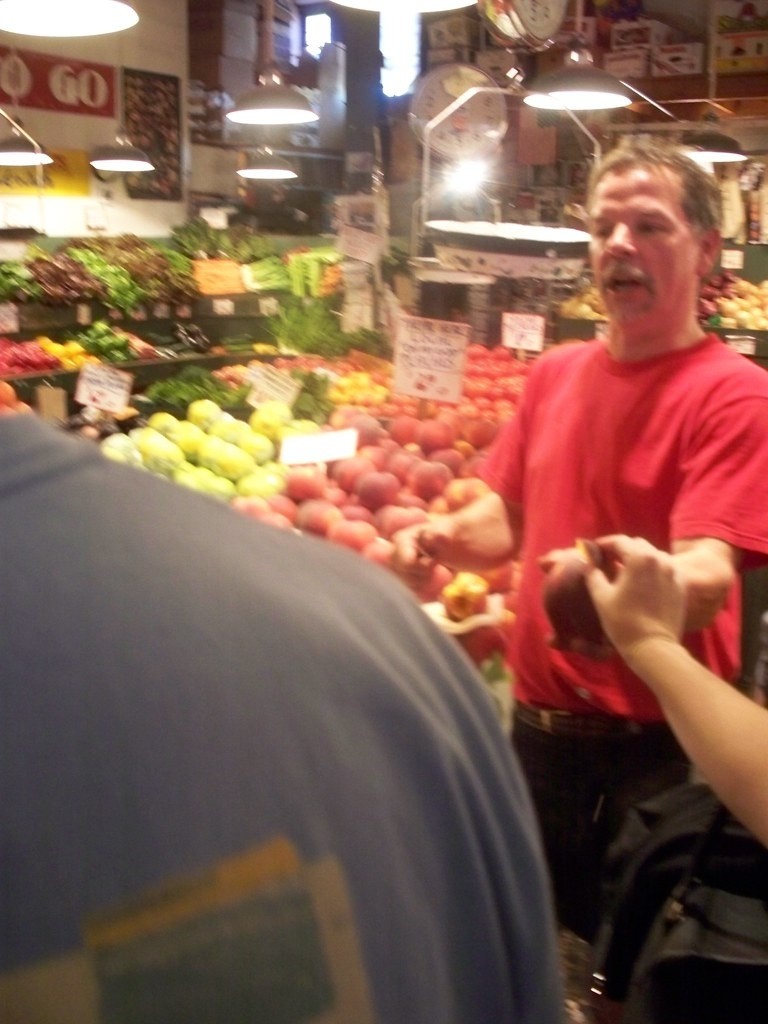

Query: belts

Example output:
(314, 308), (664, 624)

(514, 699), (669, 739)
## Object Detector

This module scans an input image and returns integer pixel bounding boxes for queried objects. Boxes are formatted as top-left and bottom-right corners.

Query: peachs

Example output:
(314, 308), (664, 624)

(575, 538), (606, 570)
(541, 556), (609, 644)
(231, 411), (503, 622)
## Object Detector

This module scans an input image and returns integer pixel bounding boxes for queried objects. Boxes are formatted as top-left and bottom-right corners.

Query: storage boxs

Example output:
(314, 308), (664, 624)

(423, 14), (768, 88)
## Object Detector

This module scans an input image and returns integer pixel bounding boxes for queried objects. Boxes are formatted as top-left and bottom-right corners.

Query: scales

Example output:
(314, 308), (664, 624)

(418, 0), (600, 281)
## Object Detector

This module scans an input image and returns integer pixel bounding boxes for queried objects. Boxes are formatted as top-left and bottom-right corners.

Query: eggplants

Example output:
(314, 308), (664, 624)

(164, 320), (210, 352)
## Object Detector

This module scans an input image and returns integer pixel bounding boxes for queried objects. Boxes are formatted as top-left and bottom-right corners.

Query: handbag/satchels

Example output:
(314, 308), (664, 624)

(591, 567), (768, 1024)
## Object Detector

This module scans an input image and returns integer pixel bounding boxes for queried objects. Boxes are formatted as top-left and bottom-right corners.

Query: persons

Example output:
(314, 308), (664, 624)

(0, 409), (562, 1024)
(398, 145), (768, 1024)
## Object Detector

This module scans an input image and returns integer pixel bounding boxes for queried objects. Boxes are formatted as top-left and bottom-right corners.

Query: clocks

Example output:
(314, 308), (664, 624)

(408, 63), (510, 163)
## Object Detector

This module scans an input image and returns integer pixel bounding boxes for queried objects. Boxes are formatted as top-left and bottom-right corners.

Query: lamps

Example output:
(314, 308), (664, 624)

(0, 117), (54, 167)
(0, 0), (139, 38)
(236, 136), (301, 180)
(90, 126), (155, 184)
(523, 37), (682, 123)
(224, 60), (320, 126)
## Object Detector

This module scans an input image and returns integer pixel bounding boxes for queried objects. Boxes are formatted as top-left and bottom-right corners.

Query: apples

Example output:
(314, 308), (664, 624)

(98, 345), (529, 493)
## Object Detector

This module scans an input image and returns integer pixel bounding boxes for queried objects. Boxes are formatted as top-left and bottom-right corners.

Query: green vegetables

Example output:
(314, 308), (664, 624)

(244, 302), (388, 356)
(1, 218), (393, 310)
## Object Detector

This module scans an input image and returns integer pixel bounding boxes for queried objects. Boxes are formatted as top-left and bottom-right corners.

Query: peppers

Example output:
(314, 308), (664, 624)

(1, 320), (140, 378)
